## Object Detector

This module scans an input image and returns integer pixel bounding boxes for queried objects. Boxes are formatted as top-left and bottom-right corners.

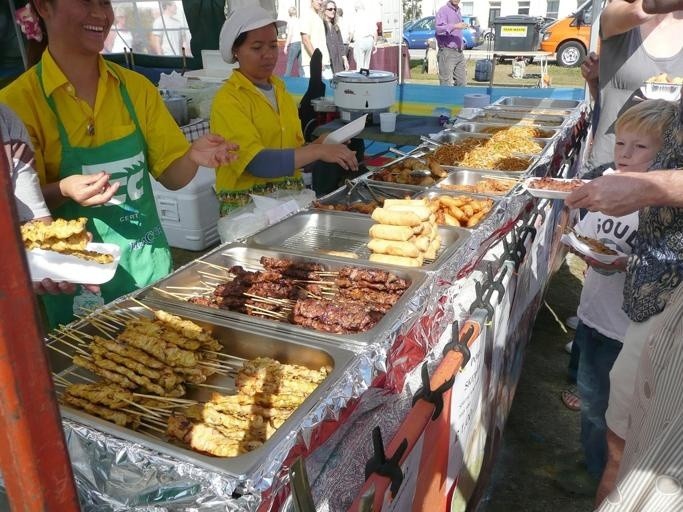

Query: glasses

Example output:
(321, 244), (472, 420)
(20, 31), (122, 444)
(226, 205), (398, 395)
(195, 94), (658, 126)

(326, 8), (336, 11)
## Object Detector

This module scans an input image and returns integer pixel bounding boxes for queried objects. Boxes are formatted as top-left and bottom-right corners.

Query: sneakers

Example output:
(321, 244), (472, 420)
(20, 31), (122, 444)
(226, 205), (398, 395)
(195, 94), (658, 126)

(555, 466), (598, 496)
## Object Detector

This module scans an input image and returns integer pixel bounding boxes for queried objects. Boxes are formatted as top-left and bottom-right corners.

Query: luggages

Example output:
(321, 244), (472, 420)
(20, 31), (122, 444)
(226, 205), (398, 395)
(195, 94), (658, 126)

(475, 35), (492, 81)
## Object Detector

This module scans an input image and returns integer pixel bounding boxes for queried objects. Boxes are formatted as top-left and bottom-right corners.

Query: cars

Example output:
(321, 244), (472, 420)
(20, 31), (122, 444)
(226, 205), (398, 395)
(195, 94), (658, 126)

(481, 28), (495, 40)
(402, 15), (485, 49)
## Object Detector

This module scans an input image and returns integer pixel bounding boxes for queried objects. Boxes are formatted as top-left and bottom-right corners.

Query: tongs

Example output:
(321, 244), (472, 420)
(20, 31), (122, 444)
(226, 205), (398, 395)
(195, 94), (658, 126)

(346, 177), (401, 204)
(346, 180), (383, 208)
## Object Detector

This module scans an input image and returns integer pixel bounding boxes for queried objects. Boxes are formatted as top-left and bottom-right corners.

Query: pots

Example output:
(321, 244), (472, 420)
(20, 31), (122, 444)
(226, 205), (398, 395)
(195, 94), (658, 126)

(158, 89), (193, 127)
(331, 67), (399, 125)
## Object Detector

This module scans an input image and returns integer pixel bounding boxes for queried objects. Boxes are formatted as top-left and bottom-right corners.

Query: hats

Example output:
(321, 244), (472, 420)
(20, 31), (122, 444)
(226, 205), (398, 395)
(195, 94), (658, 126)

(218, 6), (288, 64)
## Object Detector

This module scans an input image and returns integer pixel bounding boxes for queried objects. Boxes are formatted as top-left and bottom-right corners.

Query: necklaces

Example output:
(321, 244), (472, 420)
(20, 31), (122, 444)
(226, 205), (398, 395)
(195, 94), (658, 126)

(63, 65), (98, 136)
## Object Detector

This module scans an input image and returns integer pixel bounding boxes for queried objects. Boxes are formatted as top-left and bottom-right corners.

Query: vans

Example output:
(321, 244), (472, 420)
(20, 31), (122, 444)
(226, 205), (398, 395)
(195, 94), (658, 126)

(540, 0), (605, 67)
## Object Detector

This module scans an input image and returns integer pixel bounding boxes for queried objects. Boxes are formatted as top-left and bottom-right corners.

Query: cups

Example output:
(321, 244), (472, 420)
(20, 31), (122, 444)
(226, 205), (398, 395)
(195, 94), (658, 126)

(379, 112), (397, 133)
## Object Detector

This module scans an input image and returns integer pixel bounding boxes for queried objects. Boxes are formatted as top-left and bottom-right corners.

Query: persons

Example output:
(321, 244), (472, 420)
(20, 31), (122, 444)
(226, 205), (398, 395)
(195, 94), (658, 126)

(283, 7), (305, 78)
(0, 1), (241, 333)
(593, 285), (683, 512)
(433, 0), (471, 87)
(579, 51), (600, 139)
(150, 1), (185, 57)
(0, 102), (97, 296)
(110, 6), (133, 54)
(347, 4), (377, 70)
(209, 5), (359, 248)
(335, 8), (350, 58)
(298, 0), (333, 79)
(319, 1), (349, 74)
(561, 0), (681, 411)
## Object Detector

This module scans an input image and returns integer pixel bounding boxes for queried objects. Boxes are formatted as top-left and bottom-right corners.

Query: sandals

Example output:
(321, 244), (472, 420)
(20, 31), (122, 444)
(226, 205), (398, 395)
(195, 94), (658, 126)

(561, 385), (582, 411)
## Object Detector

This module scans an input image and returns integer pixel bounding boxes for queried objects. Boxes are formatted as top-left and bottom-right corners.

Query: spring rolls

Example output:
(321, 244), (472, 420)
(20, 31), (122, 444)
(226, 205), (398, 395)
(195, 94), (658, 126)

(366, 199), (440, 267)
(313, 249), (359, 260)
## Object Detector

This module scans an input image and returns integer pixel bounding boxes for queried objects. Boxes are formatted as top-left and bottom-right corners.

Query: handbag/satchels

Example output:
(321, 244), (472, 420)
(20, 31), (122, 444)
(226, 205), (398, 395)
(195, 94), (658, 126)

(511, 58), (527, 79)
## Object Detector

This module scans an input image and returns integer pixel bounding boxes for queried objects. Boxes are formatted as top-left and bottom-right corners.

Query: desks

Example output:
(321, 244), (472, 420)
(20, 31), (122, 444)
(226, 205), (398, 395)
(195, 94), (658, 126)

(347, 41), (411, 79)
(270, 37), (299, 75)
(463, 48), (553, 86)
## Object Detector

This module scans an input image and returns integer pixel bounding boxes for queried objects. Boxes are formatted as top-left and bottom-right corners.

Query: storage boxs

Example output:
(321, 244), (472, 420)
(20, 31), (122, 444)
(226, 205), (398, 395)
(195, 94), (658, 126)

(184, 68), (234, 81)
(201, 49), (239, 68)
(148, 164), (220, 251)
(152, 80), (220, 119)
